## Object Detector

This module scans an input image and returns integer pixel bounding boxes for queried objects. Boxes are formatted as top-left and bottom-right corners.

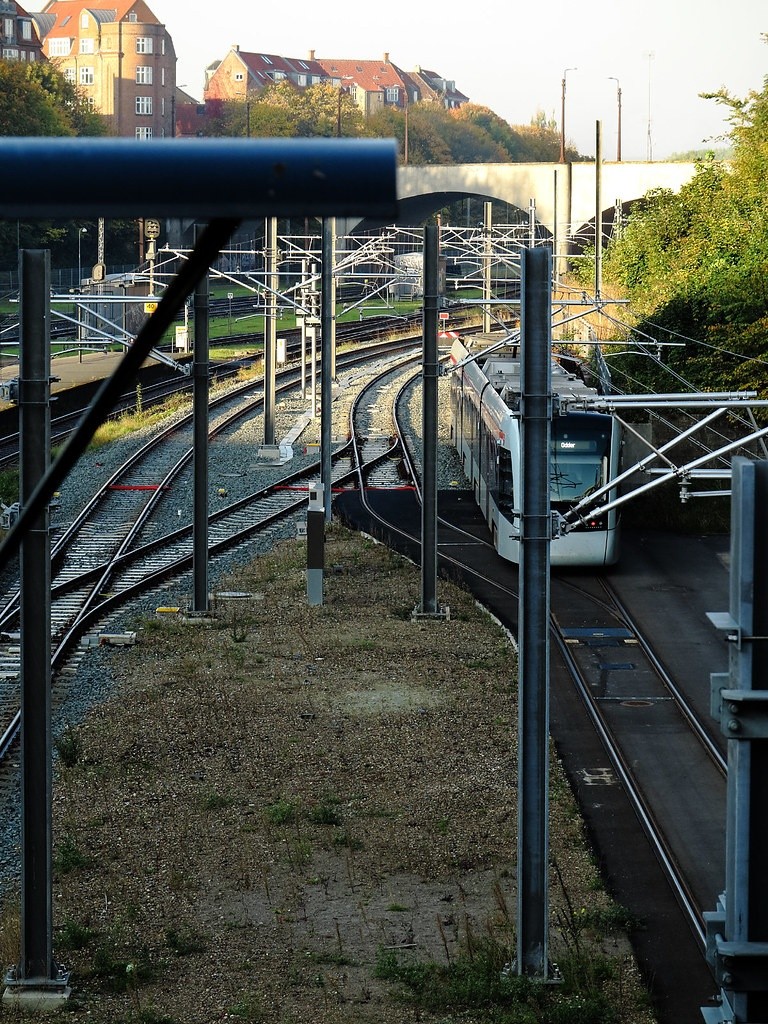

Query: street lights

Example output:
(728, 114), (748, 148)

(78, 227), (88, 363)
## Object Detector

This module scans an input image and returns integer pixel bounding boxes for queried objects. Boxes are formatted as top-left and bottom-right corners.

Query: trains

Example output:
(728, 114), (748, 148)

(450, 337), (626, 571)
